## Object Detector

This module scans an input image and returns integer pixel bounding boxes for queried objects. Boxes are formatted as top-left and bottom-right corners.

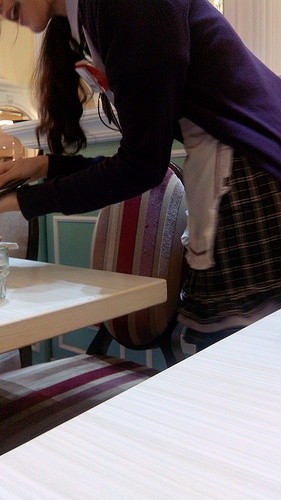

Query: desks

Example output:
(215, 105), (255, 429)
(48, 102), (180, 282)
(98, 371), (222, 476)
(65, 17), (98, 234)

(0, 250), (169, 353)
(1, 299), (281, 500)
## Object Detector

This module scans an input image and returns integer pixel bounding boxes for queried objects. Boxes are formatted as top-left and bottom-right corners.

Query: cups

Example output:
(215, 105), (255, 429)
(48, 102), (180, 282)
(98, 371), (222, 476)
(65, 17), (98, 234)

(0, 264), (10, 299)
(0, 245), (9, 269)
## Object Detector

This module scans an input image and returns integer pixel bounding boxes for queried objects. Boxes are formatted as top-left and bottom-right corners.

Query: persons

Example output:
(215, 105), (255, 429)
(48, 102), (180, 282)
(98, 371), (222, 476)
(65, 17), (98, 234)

(0, 0), (280, 363)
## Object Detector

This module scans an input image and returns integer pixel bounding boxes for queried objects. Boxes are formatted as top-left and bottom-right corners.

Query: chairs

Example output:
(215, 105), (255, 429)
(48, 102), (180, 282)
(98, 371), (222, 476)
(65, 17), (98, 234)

(0, 162), (196, 456)
(0, 192), (42, 370)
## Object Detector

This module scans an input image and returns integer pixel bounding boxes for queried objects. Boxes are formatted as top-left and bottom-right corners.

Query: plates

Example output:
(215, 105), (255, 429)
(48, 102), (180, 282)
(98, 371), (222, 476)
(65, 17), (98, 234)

(0, 178), (27, 196)
